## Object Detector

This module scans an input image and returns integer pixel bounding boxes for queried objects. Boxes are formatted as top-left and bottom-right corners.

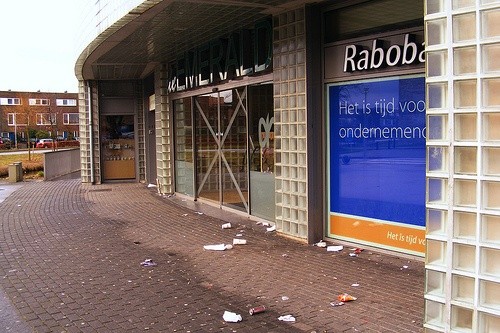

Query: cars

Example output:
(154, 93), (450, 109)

(27, 139), (39, 148)
(59, 137), (80, 147)
(0, 137), (12, 149)
(36, 138), (59, 148)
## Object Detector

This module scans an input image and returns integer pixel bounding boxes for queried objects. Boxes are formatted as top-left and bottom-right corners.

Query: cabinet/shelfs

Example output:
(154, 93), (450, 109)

(174, 96), (274, 193)
